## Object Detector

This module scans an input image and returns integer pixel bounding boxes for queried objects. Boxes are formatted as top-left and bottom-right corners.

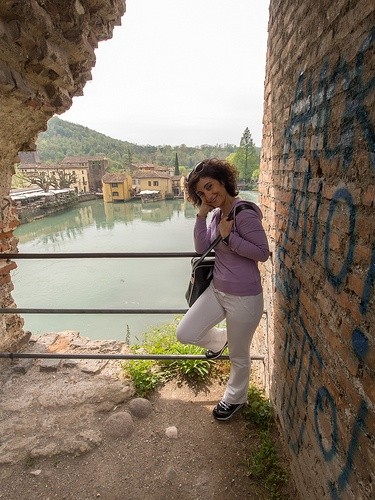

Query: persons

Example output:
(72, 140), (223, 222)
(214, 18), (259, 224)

(176, 157), (270, 421)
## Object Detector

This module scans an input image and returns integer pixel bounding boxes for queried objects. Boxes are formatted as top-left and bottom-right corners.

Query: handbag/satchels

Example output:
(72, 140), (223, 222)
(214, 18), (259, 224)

(185, 258), (215, 307)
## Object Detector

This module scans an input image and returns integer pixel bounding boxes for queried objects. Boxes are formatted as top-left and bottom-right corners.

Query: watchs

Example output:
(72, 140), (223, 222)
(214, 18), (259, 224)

(222, 233), (230, 246)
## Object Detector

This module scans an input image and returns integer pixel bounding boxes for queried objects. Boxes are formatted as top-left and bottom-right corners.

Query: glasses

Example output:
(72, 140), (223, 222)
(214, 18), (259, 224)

(184, 161), (205, 182)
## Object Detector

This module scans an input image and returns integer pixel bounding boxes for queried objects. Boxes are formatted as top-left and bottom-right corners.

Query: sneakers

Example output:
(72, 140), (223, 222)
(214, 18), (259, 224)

(212, 399), (244, 421)
(205, 340), (228, 358)
